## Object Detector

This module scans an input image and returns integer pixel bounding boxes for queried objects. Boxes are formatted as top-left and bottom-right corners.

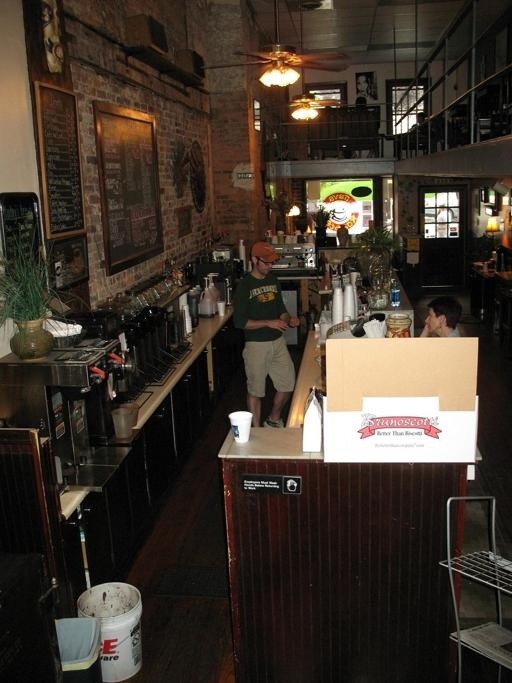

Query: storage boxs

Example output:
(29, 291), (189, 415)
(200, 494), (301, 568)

(323, 337), (480, 466)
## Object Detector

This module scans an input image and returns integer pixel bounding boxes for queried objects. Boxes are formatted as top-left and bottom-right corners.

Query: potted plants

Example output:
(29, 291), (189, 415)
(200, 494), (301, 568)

(355, 225), (405, 287)
(0, 223), (69, 362)
(265, 192), (330, 246)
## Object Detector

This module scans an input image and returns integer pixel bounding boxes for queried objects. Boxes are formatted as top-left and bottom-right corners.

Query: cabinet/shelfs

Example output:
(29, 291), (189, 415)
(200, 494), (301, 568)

(438, 495), (512, 683)
(59, 306), (246, 614)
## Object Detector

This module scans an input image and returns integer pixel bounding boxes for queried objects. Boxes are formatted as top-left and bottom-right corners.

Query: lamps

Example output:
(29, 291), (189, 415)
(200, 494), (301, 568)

(258, 44), (301, 87)
(290, 94), (319, 122)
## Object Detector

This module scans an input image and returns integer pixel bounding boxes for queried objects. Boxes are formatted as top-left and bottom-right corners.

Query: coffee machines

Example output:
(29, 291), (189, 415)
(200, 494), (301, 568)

(0, 337), (134, 492)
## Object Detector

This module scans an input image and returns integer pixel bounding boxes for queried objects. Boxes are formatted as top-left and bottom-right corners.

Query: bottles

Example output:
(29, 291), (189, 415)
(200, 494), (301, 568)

(385, 312), (412, 337)
(390, 280), (401, 307)
(318, 252), (328, 273)
(110, 402), (140, 438)
(188, 284), (202, 328)
(265, 230), (314, 245)
(110, 269), (177, 321)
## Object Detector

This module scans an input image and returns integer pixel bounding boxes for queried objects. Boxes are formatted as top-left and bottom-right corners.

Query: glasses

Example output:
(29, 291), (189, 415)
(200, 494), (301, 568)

(254, 256), (275, 266)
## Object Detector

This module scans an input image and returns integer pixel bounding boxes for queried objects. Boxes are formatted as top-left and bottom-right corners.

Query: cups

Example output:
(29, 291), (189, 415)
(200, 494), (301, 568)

(217, 301), (226, 316)
(332, 272), (361, 325)
(228, 411), (253, 443)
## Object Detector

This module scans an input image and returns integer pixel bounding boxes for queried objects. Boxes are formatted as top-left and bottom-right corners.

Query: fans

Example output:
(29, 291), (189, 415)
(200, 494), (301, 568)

(253, 66), (340, 111)
(201, 0), (346, 80)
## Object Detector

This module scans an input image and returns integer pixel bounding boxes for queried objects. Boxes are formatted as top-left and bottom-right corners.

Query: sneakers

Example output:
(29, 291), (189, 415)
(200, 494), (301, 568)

(264, 415), (284, 429)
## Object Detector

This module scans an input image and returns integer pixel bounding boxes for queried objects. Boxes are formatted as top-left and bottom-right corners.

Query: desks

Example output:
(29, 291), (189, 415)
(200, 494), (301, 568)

(469, 261), (512, 340)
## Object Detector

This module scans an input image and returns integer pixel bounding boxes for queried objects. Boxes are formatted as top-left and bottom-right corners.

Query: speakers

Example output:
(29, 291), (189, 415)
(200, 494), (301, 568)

(174, 47), (206, 79)
(124, 12), (169, 55)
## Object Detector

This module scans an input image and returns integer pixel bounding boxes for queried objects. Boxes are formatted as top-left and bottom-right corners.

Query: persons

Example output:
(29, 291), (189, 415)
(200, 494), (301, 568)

(231, 242), (300, 428)
(418, 295), (462, 337)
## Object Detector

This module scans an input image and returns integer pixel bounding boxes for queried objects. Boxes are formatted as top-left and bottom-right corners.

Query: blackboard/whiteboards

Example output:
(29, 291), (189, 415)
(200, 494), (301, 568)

(35, 81), (86, 239)
(91, 99), (165, 276)
(0, 193), (42, 275)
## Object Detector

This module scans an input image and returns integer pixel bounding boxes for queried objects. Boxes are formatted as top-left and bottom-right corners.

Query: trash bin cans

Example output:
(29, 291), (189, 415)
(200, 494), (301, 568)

(55, 617), (101, 683)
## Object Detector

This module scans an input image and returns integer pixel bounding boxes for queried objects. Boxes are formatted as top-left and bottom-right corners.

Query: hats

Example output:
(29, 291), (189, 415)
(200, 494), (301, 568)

(251, 242), (281, 262)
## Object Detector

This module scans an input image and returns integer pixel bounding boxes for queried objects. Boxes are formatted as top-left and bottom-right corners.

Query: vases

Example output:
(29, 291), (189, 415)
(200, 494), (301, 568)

(337, 225), (349, 247)
